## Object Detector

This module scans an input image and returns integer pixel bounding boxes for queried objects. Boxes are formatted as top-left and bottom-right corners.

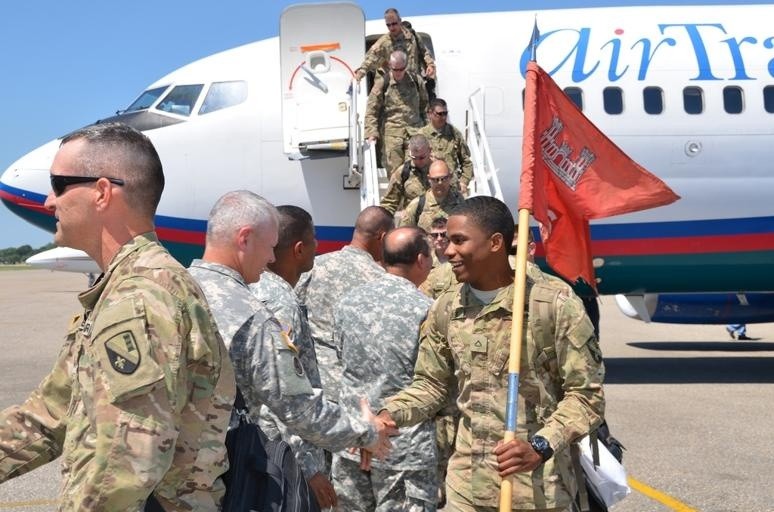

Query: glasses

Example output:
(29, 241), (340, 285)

(391, 65), (408, 72)
(509, 240), (534, 256)
(49, 173), (125, 198)
(431, 109), (449, 116)
(430, 231), (448, 238)
(386, 19), (401, 27)
(428, 175), (450, 182)
(409, 148), (430, 160)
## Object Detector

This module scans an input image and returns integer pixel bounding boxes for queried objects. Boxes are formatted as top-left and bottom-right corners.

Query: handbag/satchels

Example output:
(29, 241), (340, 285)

(221, 420), (272, 512)
(590, 419), (626, 465)
(574, 431), (634, 510)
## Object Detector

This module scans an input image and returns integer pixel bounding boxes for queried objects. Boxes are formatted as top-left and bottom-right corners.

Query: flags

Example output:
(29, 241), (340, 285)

(517, 60), (681, 296)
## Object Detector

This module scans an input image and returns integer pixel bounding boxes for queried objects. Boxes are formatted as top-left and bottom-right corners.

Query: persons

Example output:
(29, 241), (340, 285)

(364, 51), (431, 182)
(726, 323), (752, 340)
(354, 7), (436, 82)
(394, 159), (465, 236)
(1, 123), (237, 512)
(379, 134), (460, 217)
(182, 190), (605, 512)
(404, 99), (474, 195)
